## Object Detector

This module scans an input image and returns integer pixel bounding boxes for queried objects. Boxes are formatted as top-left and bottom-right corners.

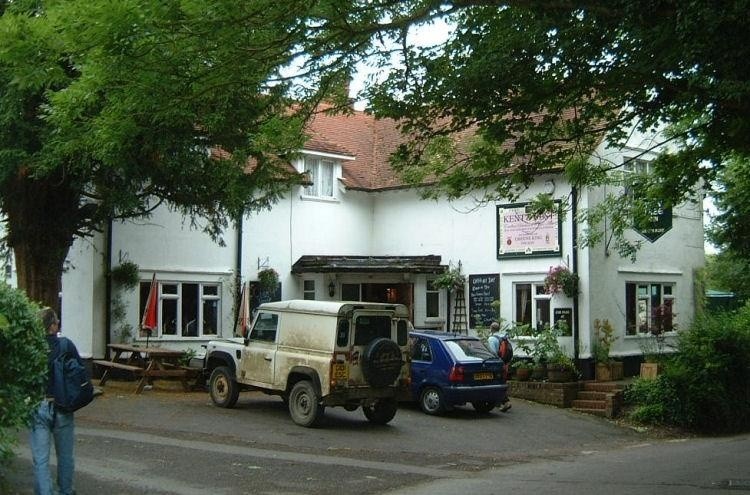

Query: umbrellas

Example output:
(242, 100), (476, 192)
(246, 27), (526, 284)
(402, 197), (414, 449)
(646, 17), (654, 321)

(235, 281), (251, 338)
(141, 272), (158, 371)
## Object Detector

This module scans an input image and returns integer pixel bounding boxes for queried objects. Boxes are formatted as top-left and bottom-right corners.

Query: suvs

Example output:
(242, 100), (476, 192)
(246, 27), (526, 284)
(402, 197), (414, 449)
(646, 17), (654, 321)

(396, 328), (505, 417)
(200, 298), (414, 428)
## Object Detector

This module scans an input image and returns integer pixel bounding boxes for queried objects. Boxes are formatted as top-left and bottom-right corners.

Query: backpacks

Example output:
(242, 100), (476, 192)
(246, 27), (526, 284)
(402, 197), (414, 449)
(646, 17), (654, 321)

(52, 337), (95, 414)
(487, 334), (515, 363)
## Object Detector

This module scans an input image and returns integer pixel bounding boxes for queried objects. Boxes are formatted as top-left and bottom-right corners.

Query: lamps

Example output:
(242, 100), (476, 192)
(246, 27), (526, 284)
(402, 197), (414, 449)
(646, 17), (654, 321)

(327, 279), (336, 298)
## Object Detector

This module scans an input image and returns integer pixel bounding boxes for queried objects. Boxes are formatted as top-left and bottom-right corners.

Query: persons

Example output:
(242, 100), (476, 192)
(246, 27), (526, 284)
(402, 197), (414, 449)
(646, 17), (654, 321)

(487, 322), (512, 412)
(30, 306), (85, 495)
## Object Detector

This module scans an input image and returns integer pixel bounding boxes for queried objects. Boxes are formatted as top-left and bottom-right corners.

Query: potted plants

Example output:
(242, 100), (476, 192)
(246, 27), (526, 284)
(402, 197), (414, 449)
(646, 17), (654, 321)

(632, 321), (678, 382)
(592, 315), (626, 383)
(476, 299), (584, 384)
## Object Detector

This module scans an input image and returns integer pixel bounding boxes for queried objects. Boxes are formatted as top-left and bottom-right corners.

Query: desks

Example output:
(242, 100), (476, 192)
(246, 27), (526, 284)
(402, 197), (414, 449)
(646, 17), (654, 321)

(99, 341), (196, 396)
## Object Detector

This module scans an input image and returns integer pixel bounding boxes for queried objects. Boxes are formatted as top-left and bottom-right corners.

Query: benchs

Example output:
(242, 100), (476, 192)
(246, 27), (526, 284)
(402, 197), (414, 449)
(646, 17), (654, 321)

(157, 360), (206, 375)
(91, 358), (144, 377)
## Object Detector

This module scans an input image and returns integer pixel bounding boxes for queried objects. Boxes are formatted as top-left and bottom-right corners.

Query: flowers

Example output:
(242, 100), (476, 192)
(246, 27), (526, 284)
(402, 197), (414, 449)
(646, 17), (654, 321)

(541, 261), (583, 301)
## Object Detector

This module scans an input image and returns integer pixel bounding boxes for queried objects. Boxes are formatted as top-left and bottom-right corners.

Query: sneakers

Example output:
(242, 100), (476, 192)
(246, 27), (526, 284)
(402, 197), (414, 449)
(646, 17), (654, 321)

(499, 400), (513, 412)
